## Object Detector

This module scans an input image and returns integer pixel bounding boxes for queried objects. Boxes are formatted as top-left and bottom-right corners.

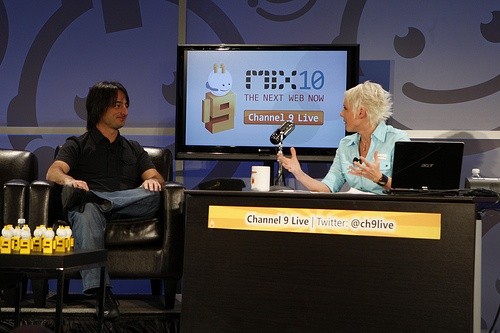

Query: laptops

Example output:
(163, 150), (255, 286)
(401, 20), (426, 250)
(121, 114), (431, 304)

(383, 141), (465, 195)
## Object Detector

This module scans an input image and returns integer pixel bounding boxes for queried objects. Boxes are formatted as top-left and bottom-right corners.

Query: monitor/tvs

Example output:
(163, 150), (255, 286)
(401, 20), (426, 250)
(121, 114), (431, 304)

(176, 42), (360, 164)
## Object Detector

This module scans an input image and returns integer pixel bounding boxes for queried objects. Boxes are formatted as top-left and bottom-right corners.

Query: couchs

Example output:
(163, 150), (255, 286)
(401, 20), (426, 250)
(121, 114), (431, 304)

(29, 145), (186, 310)
(0, 149), (39, 236)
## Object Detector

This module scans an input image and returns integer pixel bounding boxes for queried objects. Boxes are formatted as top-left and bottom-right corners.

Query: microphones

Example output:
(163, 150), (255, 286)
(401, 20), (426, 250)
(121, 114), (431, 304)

(270, 121), (295, 144)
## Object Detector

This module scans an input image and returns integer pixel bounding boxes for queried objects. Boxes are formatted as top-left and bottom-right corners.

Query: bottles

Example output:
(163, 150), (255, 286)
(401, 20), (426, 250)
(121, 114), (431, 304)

(471, 169), (481, 178)
(2, 219), (72, 254)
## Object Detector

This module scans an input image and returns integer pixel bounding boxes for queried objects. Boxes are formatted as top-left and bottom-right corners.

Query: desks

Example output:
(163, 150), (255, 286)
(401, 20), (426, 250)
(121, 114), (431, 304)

(178, 185), (500, 333)
(0, 248), (108, 333)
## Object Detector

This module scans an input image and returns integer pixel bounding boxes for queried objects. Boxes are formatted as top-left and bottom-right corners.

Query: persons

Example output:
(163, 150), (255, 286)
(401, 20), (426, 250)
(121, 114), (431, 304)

(45, 80), (164, 332)
(276, 80), (411, 195)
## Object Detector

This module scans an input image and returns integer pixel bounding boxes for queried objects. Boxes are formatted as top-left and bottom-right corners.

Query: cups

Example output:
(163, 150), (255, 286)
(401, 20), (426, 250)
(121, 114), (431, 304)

(251, 166), (270, 192)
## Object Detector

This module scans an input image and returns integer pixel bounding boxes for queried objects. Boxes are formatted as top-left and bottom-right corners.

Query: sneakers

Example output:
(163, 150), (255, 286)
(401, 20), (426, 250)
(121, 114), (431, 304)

(61, 184), (87, 213)
(95, 288), (120, 319)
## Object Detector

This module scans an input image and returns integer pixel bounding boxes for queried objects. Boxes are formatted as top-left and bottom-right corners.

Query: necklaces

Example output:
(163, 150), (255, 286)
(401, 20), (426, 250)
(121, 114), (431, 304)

(361, 137), (371, 152)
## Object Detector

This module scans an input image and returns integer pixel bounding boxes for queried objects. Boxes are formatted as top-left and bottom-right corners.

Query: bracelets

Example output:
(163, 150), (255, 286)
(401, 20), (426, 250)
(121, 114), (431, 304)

(373, 173), (389, 187)
